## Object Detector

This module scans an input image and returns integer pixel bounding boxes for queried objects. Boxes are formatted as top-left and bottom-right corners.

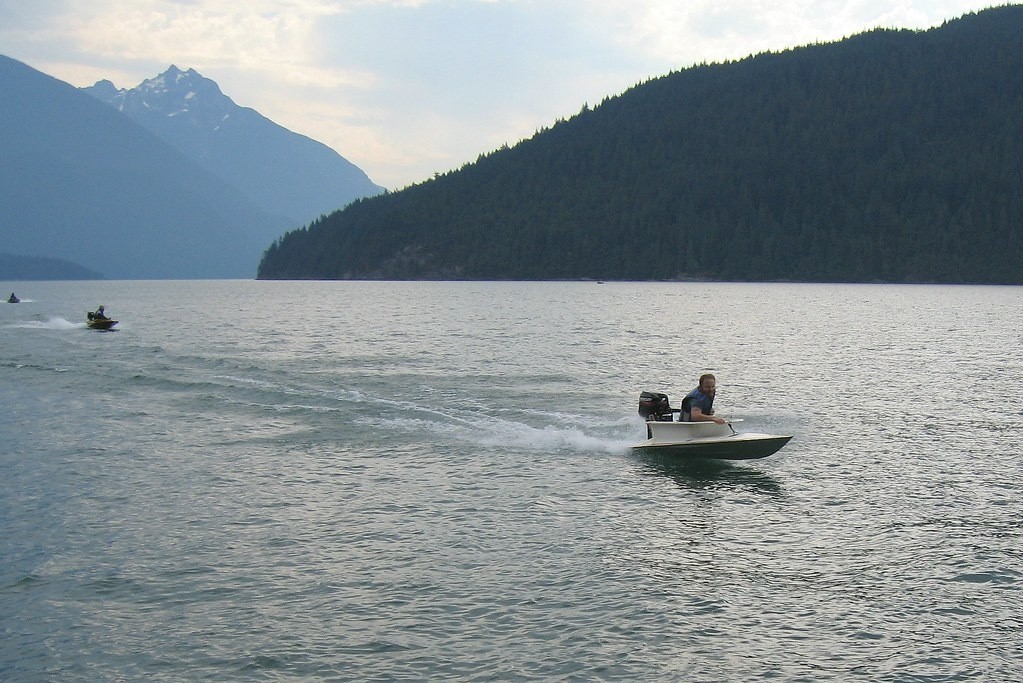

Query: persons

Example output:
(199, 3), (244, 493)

(93, 305), (111, 321)
(11, 292), (20, 302)
(676, 374), (726, 425)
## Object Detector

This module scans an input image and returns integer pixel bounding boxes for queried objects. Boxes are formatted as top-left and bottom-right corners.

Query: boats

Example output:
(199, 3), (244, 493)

(628, 390), (794, 459)
(8, 298), (20, 303)
(85, 312), (120, 330)
